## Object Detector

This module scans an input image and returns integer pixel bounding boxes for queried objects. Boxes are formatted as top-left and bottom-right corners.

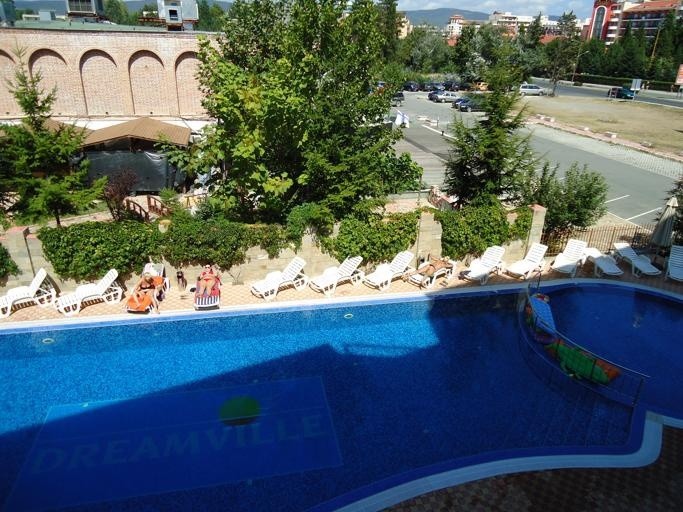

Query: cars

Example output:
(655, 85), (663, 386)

(606, 86), (636, 100)
(367, 72), (552, 112)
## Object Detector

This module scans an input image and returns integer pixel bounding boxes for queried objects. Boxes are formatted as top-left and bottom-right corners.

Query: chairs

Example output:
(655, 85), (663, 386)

(309, 254), (365, 295)
(125, 263), (169, 315)
(251, 255), (308, 302)
(193, 270), (220, 310)
(664, 245), (683, 282)
(0, 268), (123, 323)
(458, 245), (506, 286)
(548, 238), (660, 278)
(403, 257), (457, 290)
(364, 250), (416, 291)
(505, 242), (548, 281)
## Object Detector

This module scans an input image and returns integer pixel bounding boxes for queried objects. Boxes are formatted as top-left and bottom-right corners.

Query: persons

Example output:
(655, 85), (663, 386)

(131, 273), (159, 314)
(199, 263), (218, 298)
(401, 255), (454, 287)
